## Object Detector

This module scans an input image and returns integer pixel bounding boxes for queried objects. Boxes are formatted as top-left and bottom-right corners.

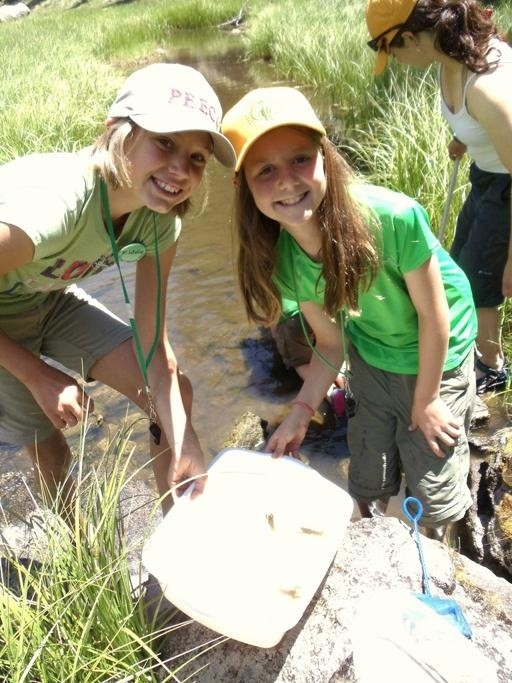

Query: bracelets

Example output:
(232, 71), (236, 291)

(290, 396), (316, 420)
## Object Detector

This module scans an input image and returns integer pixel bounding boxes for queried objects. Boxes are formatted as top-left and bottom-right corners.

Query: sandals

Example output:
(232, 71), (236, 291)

(474, 359), (509, 394)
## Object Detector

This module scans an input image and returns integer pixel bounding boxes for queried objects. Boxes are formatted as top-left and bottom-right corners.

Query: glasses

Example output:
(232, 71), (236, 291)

(367, 23), (405, 51)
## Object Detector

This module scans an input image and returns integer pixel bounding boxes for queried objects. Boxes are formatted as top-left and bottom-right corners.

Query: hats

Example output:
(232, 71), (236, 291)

(221, 87), (326, 172)
(366, 0), (418, 75)
(108, 64), (236, 168)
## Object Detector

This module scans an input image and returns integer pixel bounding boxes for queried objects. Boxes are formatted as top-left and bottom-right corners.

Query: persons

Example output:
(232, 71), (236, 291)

(363, 0), (510, 397)
(0, 62), (237, 552)
(220, 83), (479, 543)
